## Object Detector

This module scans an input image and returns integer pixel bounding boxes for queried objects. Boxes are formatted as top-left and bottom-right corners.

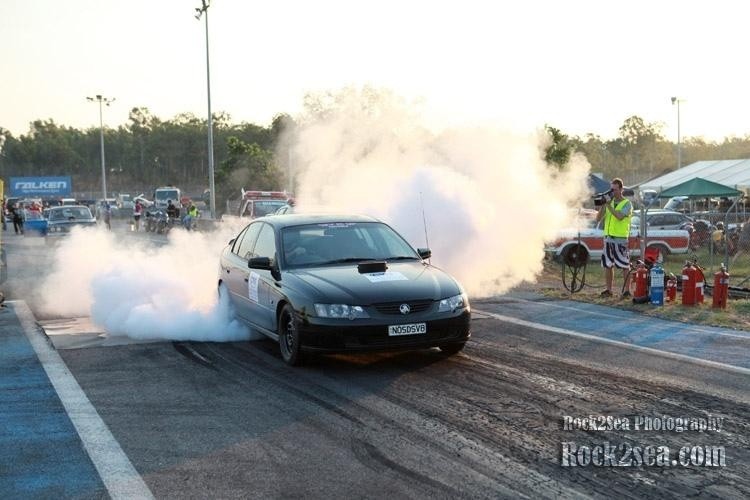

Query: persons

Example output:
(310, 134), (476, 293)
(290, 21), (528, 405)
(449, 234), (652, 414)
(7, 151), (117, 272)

(595, 177), (633, 301)
(281, 233), (315, 266)
(0, 196), (197, 237)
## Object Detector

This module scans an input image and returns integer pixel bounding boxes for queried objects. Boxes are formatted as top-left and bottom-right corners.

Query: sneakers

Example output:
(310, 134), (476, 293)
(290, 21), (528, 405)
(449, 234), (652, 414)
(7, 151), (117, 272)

(600, 290), (613, 298)
(623, 291), (632, 297)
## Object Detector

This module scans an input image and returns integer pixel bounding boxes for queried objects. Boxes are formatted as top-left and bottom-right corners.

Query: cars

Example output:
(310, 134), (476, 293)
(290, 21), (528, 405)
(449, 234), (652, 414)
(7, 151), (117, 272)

(217, 212), (471, 366)
(4, 194), (134, 251)
(542, 191), (711, 268)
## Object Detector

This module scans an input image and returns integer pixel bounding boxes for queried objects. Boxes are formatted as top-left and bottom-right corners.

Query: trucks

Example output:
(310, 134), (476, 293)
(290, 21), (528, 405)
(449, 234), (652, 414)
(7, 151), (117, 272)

(155, 186), (182, 218)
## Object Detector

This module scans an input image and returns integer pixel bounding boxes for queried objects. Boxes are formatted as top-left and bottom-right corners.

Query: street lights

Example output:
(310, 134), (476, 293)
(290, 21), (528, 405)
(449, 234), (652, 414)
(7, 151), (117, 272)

(671, 96), (681, 170)
(193, 0), (216, 220)
(86, 95), (115, 202)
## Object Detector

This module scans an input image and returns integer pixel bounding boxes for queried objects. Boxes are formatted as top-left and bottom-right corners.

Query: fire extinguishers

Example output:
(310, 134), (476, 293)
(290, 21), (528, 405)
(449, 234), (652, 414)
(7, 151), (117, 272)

(650, 261), (665, 306)
(681, 260), (697, 305)
(712, 262), (730, 309)
(633, 260), (649, 299)
(692, 260), (706, 305)
(666, 272), (677, 302)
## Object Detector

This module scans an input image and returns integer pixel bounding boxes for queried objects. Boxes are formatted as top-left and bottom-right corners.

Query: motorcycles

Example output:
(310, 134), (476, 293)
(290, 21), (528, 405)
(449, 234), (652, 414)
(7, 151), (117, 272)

(144, 203), (170, 234)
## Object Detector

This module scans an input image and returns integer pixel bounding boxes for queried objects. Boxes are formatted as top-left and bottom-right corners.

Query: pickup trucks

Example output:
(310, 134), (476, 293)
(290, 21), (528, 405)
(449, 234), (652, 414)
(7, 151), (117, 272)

(221, 191), (297, 234)
